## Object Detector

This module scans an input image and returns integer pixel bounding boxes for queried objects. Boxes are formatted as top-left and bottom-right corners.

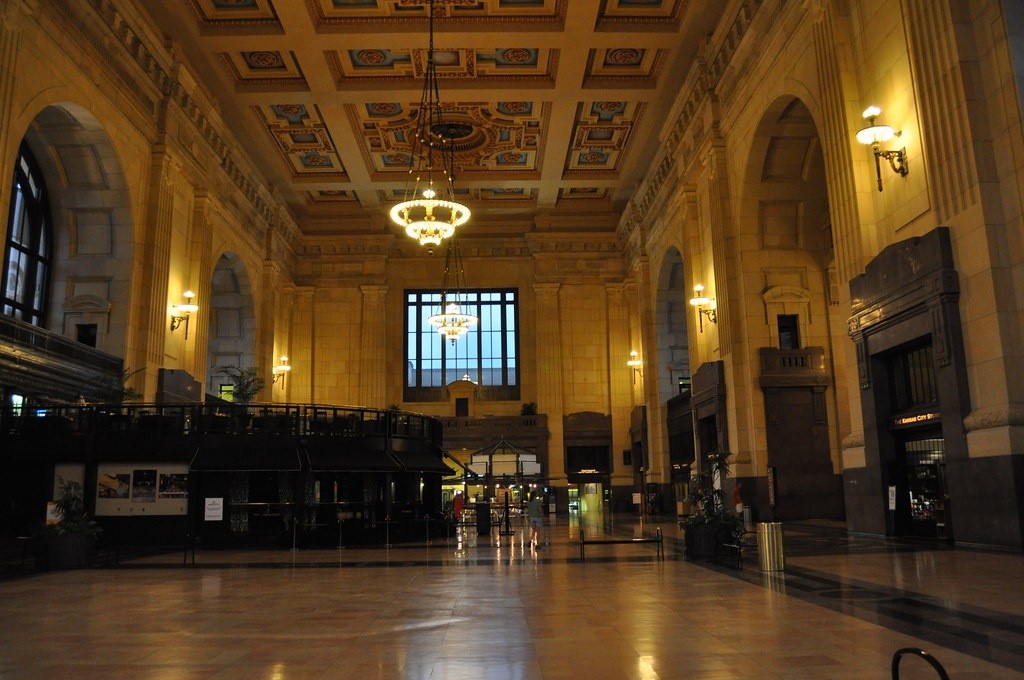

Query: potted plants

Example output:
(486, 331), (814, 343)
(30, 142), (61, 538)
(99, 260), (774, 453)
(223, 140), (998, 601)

(17, 476), (103, 571)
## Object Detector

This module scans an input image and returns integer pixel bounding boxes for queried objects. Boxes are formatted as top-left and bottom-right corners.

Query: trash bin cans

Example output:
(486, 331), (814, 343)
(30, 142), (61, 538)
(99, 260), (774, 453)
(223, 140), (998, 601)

(756, 522), (785, 572)
(743, 506), (755, 525)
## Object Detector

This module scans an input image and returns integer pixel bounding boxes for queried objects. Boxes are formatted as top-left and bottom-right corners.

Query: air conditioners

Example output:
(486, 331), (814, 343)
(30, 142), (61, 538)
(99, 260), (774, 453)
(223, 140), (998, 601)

(679, 446), (745, 561)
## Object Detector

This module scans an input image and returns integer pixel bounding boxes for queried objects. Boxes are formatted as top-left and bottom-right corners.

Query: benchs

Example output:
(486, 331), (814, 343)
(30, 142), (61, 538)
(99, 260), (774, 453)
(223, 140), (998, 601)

(580, 527), (665, 562)
(713, 531), (758, 575)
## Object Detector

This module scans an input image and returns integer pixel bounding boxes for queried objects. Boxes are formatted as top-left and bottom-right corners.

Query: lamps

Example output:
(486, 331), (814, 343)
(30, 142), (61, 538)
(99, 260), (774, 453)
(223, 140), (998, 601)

(856, 107), (906, 192)
(172, 291), (197, 340)
(388, 0), (471, 250)
(428, 129), (478, 347)
(272, 356), (292, 392)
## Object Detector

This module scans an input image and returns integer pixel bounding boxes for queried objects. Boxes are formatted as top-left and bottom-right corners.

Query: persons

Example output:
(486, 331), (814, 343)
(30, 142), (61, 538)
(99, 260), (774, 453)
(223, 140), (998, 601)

(527, 491), (545, 548)
